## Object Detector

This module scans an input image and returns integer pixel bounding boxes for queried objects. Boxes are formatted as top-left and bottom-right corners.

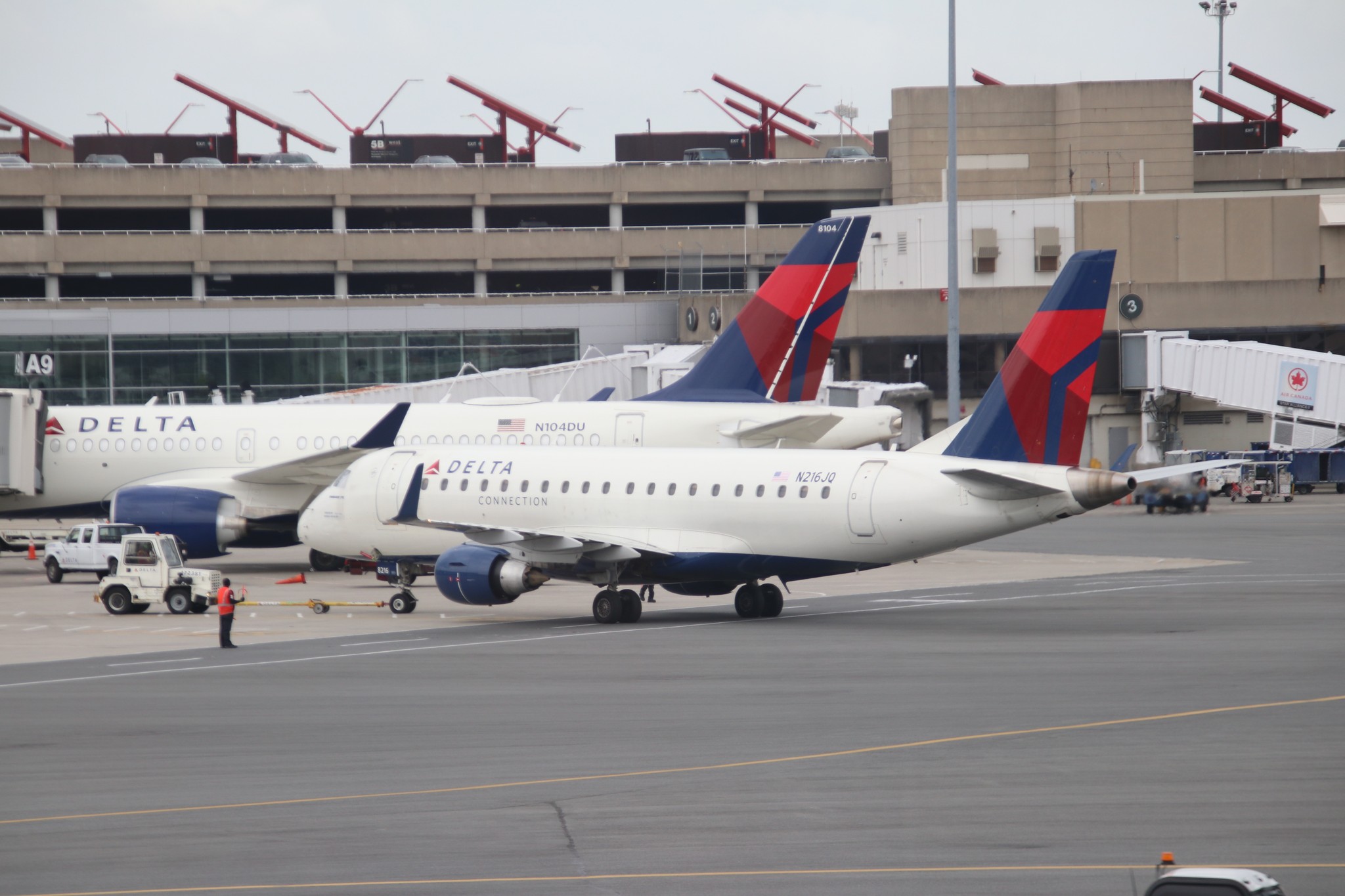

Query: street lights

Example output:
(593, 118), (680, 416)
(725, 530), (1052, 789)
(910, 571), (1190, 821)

(1200, 0), (1236, 123)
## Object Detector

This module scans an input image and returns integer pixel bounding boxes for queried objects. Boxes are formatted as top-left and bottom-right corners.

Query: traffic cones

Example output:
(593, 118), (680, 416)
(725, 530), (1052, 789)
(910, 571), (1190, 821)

(275, 572), (306, 584)
(25, 539), (39, 560)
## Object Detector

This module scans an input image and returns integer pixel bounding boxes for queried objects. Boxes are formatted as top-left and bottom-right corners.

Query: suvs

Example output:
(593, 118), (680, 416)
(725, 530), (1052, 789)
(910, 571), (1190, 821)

(2, 146), (877, 167)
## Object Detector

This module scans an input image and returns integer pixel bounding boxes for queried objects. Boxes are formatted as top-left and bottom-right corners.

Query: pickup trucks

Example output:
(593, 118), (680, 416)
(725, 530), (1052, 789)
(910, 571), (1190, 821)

(43, 524), (188, 583)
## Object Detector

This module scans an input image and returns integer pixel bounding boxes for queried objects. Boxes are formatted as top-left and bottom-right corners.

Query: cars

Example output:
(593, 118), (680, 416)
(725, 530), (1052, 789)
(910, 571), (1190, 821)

(98, 533), (224, 616)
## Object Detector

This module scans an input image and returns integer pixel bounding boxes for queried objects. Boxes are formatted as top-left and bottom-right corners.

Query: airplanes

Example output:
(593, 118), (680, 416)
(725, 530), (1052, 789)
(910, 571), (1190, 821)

(0, 215), (902, 570)
(297, 248), (1255, 626)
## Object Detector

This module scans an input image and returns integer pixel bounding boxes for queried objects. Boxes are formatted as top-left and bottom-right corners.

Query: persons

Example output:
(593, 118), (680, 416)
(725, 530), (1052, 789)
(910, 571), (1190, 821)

(217, 577), (245, 648)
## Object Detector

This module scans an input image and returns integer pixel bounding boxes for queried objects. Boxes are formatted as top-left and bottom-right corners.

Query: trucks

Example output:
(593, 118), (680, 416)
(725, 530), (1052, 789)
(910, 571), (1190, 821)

(1142, 448), (1345, 514)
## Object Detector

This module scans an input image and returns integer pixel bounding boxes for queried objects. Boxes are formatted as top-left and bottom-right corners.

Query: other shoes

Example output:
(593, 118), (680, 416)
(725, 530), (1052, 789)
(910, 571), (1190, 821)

(220, 643), (237, 648)
